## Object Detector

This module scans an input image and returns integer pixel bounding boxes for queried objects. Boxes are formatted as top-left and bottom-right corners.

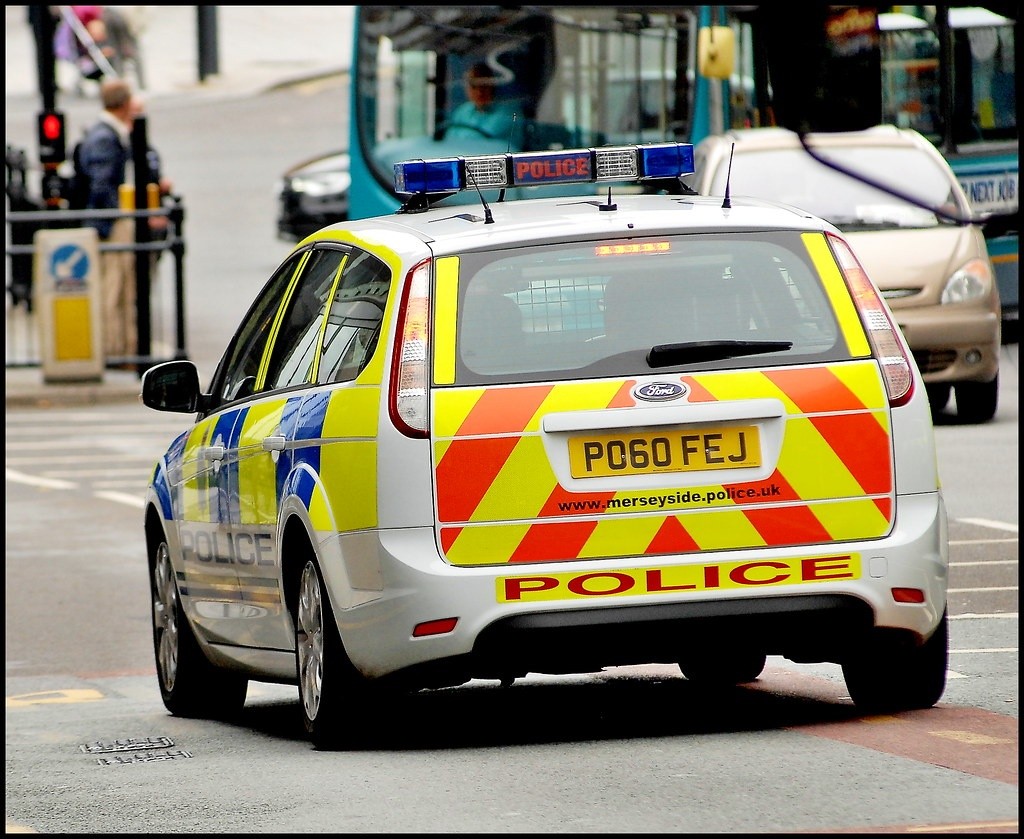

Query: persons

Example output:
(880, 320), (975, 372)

(23, 5), (175, 370)
(442, 61), (527, 147)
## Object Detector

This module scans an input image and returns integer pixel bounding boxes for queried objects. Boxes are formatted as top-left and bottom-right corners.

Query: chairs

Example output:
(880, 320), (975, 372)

(651, 272), (752, 331)
(460, 291), (523, 375)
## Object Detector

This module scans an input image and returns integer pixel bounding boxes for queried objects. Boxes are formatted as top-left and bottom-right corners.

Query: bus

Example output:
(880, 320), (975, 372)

(338, 2), (1024, 337)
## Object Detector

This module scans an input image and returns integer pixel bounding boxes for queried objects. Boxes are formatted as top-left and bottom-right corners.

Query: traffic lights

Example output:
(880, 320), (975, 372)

(36, 110), (65, 162)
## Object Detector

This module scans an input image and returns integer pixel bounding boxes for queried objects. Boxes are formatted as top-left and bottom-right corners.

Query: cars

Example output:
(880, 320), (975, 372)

(130, 140), (952, 750)
(691, 123), (1004, 425)
(271, 146), (354, 250)
